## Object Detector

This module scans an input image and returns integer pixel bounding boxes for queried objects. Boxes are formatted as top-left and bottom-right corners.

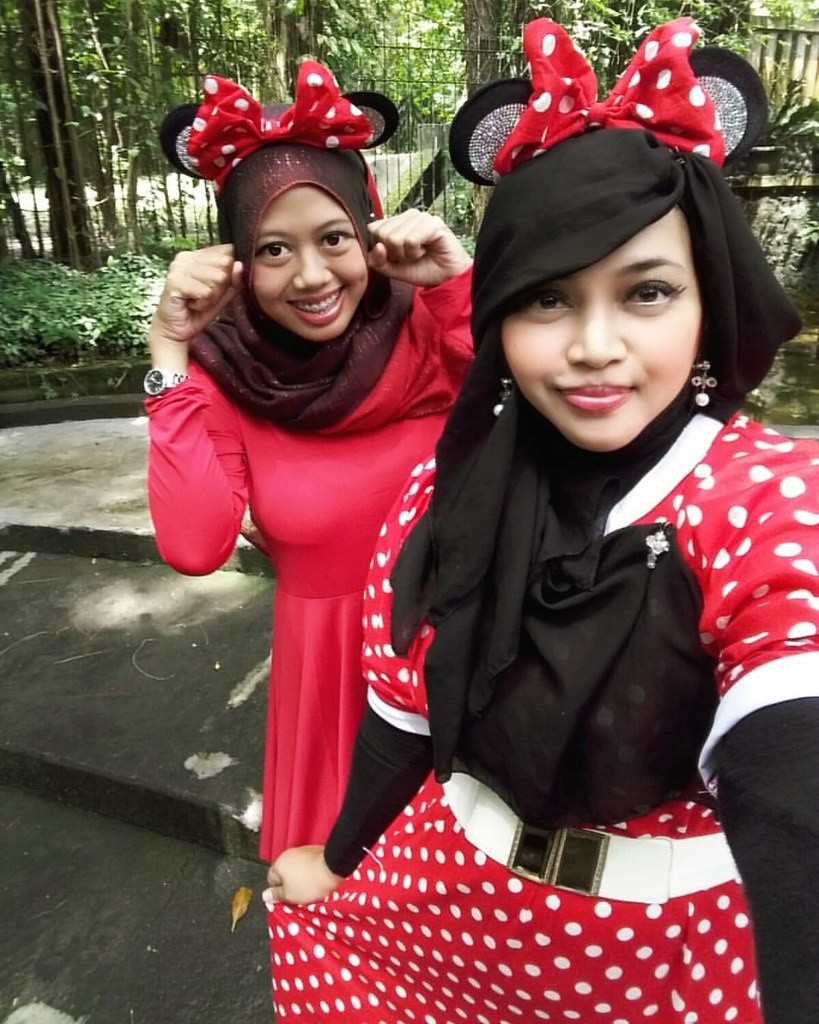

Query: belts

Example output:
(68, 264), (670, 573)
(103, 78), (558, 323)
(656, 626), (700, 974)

(442, 755), (739, 904)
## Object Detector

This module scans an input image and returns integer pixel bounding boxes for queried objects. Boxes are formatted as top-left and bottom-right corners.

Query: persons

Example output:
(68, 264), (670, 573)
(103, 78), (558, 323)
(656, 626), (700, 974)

(253, 16), (819, 1024)
(143, 60), (477, 864)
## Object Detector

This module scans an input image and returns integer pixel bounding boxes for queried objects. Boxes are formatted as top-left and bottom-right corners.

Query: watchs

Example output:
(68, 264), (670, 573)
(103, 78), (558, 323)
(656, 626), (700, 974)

(143, 368), (191, 395)
